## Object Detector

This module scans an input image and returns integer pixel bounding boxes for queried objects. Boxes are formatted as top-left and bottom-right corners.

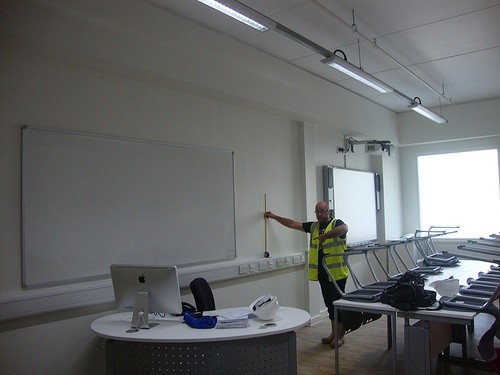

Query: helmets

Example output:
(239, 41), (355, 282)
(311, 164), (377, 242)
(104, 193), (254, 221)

(249, 293), (279, 320)
(429, 276), (459, 300)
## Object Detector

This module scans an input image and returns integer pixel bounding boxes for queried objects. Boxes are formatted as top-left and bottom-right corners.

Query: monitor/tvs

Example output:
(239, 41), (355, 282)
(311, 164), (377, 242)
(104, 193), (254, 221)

(109, 263), (183, 329)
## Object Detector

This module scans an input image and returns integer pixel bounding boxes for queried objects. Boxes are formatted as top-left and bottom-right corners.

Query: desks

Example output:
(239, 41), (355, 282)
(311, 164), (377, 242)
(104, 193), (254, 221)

(91, 307), (311, 375)
(331, 258), (500, 375)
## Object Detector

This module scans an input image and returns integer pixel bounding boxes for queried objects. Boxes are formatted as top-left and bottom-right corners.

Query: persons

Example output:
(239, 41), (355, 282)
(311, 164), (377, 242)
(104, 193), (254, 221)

(264, 202), (349, 348)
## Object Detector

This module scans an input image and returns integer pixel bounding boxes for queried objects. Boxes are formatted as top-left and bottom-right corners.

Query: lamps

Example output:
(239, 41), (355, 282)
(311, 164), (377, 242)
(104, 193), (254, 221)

(319, 55), (393, 94)
(199, 0), (277, 33)
(407, 103), (446, 124)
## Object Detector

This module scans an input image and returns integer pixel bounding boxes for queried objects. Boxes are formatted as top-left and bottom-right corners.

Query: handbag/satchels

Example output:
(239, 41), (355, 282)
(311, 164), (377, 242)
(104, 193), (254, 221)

(391, 283), (436, 313)
(378, 269), (425, 304)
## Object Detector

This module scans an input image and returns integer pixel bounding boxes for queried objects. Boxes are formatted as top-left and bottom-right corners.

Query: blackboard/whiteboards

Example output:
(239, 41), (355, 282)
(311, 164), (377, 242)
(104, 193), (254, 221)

(21, 124), (237, 288)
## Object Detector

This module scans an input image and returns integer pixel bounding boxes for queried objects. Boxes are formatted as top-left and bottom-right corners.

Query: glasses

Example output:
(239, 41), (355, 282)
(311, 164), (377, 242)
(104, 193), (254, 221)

(313, 209), (329, 215)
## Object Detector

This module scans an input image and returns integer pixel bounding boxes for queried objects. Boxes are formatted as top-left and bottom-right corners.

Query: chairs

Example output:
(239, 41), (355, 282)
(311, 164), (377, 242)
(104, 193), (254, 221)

(322, 226), (500, 362)
(190, 278), (215, 311)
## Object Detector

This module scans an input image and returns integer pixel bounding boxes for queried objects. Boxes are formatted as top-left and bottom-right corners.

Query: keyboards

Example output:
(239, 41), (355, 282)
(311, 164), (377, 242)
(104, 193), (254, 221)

(147, 312), (184, 322)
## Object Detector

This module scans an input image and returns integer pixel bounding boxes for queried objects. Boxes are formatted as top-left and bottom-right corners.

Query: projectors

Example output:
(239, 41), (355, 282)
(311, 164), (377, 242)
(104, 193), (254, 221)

(364, 143), (397, 156)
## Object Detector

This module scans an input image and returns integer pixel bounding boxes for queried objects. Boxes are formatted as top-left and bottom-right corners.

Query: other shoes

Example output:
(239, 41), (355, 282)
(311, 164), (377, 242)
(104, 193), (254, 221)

(321, 333), (334, 344)
(330, 337), (345, 348)
